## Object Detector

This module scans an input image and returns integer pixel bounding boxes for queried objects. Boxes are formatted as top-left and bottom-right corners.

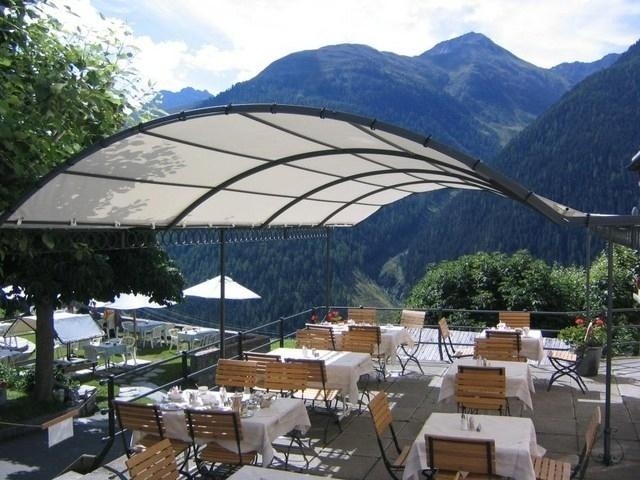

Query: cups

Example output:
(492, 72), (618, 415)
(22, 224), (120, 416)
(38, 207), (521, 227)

(310, 306), (393, 333)
(153, 384), (275, 418)
(487, 320), (529, 337)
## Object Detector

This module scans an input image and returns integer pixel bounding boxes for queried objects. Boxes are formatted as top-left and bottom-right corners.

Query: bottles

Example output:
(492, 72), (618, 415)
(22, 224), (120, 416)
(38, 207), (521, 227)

(460, 406), (476, 431)
(476, 355), (491, 368)
(301, 345), (320, 358)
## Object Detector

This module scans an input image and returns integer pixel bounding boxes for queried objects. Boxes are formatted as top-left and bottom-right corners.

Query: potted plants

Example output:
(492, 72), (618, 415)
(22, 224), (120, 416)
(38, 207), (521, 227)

(563, 326), (603, 378)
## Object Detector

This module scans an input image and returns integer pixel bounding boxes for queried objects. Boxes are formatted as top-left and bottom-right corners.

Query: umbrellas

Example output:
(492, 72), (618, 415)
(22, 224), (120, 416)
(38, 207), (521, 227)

(88, 290), (178, 338)
(182, 275), (262, 301)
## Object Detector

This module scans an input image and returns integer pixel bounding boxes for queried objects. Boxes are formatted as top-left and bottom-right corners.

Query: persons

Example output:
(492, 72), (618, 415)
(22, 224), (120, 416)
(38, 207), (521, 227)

(103, 322), (116, 339)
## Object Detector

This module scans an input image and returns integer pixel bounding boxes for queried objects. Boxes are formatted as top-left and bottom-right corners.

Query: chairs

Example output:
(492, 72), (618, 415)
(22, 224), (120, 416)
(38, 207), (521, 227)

(531, 406), (600, 479)
(215, 358), (257, 393)
(84, 320), (219, 373)
(349, 308), (375, 324)
(125, 438), (193, 479)
(111, 399), (194, 473)
(395, 310), (426, 377)
(369, 391), (430, 480)
(242, 324), (387, 446)
(184, 408), (258, 479)
(546, 322), (592, 396)
(425, 433), (510, 479)
(438, 311), (530, 416)
